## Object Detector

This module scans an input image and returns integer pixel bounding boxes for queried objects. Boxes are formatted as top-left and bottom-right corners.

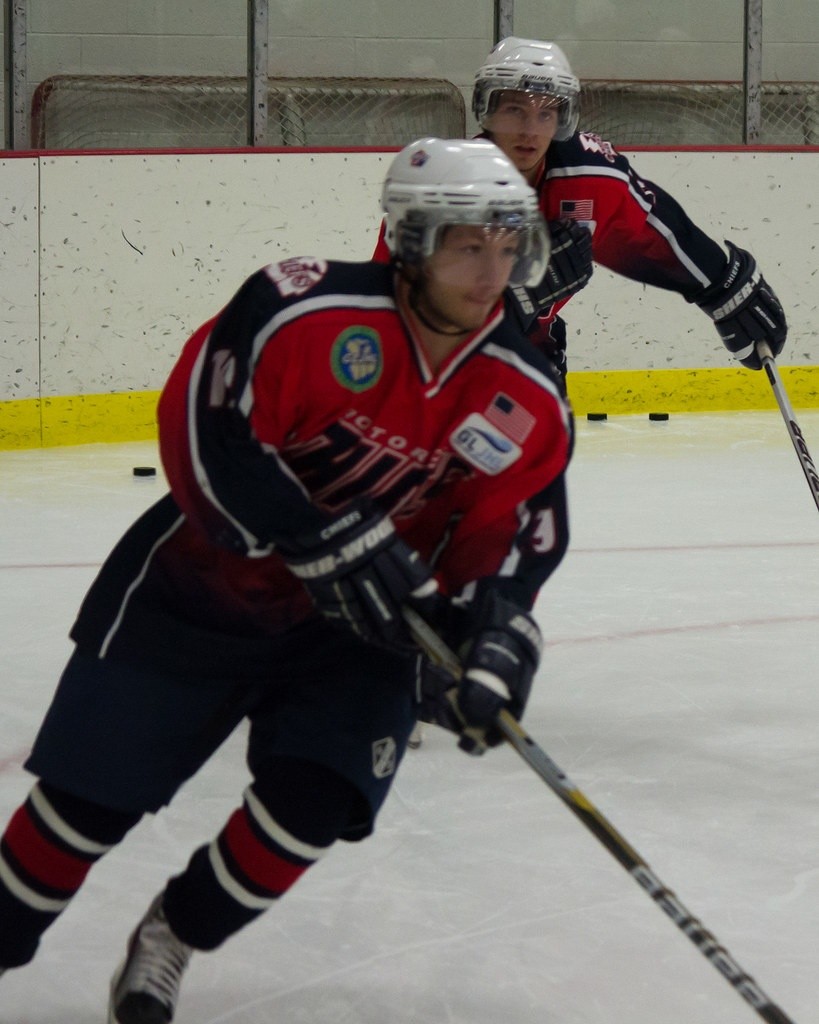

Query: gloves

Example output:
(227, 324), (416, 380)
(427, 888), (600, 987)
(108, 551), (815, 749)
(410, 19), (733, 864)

(699, 238), (788, 370)
(283, 507), (446, 646)
(422, 598), (543, 756)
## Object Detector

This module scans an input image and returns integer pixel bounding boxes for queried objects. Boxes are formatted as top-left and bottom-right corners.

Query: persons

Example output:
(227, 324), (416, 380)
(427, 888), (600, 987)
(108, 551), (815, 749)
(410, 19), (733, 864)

(372, 38), (786, 746)
(0, 137), (570, 1022)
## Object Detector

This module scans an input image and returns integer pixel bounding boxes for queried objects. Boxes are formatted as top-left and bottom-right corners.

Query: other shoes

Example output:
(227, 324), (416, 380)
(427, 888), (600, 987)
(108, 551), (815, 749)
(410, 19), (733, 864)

(115, 894), (191, 1023)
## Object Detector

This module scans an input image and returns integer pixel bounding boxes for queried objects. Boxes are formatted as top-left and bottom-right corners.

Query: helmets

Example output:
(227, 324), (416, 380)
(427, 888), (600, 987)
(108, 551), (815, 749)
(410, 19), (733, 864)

(471, 36), (580, 141)
(380, 135), (551, 288)
